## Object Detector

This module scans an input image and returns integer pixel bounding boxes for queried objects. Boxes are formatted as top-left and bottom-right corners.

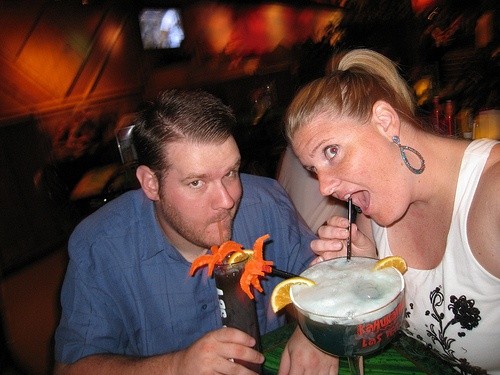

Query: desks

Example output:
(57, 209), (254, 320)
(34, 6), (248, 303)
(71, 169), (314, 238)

(260, 319), (454, 375)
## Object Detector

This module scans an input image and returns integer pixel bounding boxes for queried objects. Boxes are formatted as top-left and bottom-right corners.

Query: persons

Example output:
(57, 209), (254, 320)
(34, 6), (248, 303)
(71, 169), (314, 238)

(285, 48), (500, 375)
(54, 88), (340, 375)
(277, 0), (500, 236)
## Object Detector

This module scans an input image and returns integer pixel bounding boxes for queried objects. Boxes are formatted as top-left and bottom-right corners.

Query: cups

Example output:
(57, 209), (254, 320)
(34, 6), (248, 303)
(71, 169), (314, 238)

(456, 108), (473, 138)
(213, 252), (262, 375)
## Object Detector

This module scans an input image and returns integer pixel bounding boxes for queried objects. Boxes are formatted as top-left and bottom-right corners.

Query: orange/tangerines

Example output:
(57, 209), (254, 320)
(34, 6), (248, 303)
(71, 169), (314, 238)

(375, 256), (407, 274)
(227, 250), (253, 269)
(270, 277), (314, 313)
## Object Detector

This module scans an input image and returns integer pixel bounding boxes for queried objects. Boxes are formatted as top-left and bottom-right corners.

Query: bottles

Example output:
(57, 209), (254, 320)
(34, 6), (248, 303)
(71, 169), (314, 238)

(431, 96), (442, 132)
(441, 100), (455, 136)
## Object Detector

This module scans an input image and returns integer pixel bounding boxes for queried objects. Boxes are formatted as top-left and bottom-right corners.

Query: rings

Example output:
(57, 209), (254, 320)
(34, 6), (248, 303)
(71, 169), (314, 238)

(319, 257), (324, 262)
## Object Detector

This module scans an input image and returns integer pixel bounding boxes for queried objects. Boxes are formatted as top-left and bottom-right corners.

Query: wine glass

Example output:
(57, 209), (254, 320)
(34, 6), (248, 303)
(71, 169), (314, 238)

(289, 255), (406, 375)
